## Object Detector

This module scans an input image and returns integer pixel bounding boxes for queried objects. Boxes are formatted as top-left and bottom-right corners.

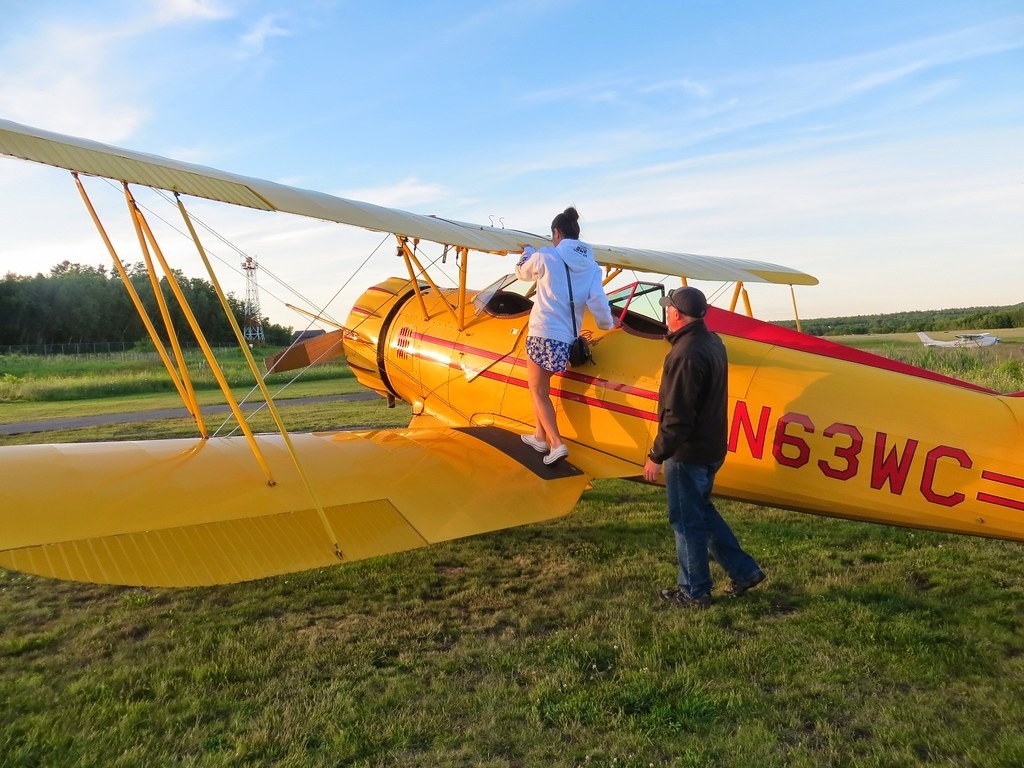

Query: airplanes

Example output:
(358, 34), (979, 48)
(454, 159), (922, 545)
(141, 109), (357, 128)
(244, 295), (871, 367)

(2, 114), (1023, 586)
(915, 331), (999, 350)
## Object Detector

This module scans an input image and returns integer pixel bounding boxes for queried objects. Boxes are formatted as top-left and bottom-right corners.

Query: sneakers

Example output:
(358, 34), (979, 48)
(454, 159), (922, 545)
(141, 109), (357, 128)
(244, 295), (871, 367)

(661, 590), (711, 608)
(521, 434), (547, 452)
(724, 571), (766, 598)
(543, 444), (568, 465)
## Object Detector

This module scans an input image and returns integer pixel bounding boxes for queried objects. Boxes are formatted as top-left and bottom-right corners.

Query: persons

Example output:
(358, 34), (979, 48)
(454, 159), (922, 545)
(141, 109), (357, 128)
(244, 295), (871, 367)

(642, 286), (765, 607)
(514, 207), (621, 465)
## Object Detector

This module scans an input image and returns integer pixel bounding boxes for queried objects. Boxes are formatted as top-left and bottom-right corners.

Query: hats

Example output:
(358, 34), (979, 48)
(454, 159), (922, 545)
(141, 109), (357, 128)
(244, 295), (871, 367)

(659, 287), (707, 318)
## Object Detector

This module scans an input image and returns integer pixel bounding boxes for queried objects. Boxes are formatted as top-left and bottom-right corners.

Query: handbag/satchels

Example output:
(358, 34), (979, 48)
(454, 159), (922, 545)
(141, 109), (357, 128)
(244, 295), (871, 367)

(570, 337), (597, 367)
